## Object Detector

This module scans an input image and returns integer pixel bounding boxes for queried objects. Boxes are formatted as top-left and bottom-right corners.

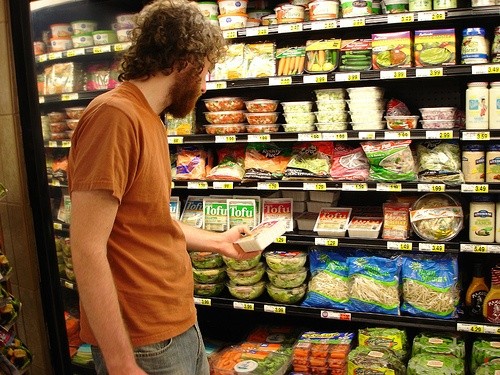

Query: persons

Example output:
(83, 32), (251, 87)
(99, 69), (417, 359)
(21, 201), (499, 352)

(66, 0), (267, 375)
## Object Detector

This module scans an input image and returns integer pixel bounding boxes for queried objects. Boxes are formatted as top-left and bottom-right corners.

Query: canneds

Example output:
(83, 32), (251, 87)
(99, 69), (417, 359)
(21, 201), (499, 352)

(466, 82), (500, 129)
(461, 27), (491, 64)
(491, 26), (500, 63)
